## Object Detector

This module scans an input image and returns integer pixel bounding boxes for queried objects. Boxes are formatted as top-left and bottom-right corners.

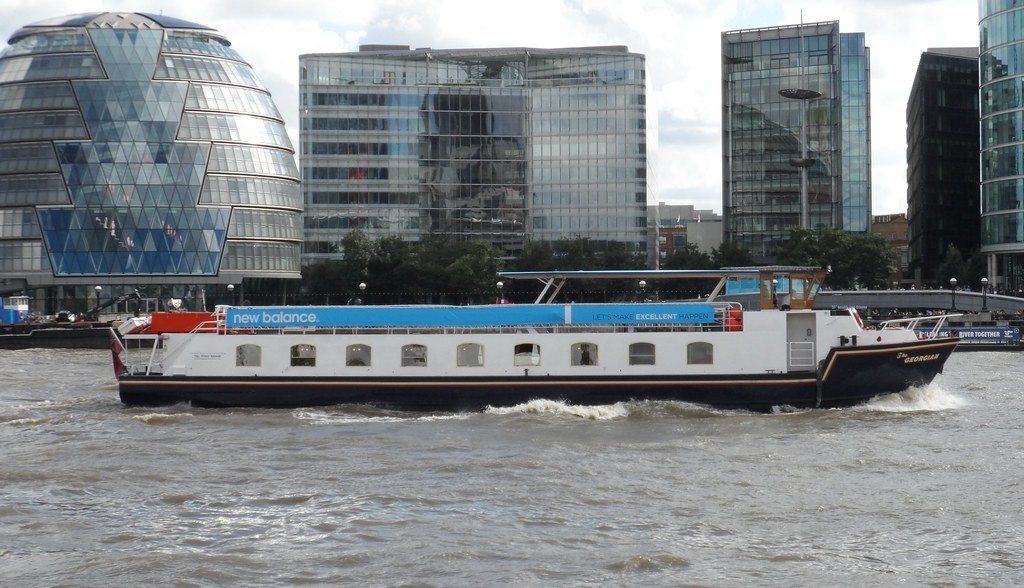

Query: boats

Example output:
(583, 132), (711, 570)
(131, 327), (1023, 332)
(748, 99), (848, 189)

(107, 263), (963, 413)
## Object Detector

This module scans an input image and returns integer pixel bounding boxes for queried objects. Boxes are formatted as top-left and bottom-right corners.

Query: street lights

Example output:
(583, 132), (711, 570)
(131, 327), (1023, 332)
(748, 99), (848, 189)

(639, 279), (647, 303)
(228, 283), (235, 307)
(95, 286), (103, 321)
(981, 277), (989, 312)
(949, 278), (958, 314)
(359, 283), (366, 306)
(496, 281), (504, 304)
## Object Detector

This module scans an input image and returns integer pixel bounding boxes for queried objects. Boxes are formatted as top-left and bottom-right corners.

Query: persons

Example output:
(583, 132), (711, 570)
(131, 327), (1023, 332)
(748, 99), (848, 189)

(819, 284), (1024, 320)
(782, 290), (796, 310)
